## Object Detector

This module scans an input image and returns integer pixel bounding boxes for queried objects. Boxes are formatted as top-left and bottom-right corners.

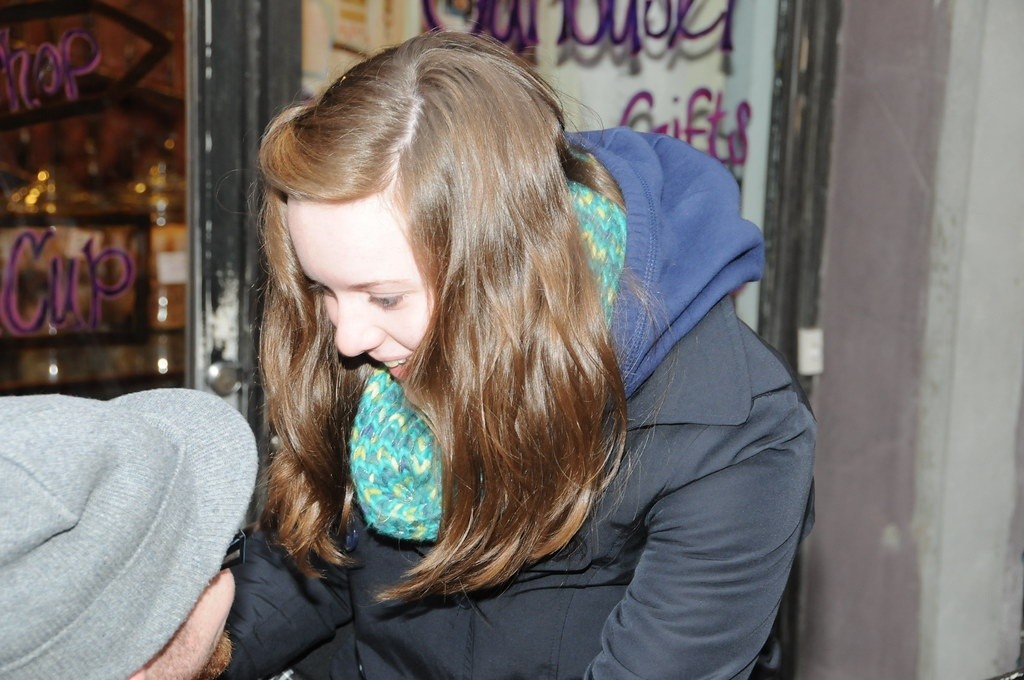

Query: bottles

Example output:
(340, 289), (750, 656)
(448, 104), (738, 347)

(145, 145), (193, 331)
(0, 226), (146, 334)
(0, 334), (188, 385)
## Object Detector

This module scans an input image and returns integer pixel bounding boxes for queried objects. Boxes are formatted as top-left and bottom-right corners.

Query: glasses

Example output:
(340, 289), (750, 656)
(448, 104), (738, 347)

(220, 529), (245, 572)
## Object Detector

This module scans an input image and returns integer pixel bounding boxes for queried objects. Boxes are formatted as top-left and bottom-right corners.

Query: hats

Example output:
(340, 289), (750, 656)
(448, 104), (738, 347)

(0, 388), (258, 680)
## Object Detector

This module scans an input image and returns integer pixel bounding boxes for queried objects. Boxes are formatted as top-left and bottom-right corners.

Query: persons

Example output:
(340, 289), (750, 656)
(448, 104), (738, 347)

(217, 31), (815, 680)
(0, 387), (259, 680)
